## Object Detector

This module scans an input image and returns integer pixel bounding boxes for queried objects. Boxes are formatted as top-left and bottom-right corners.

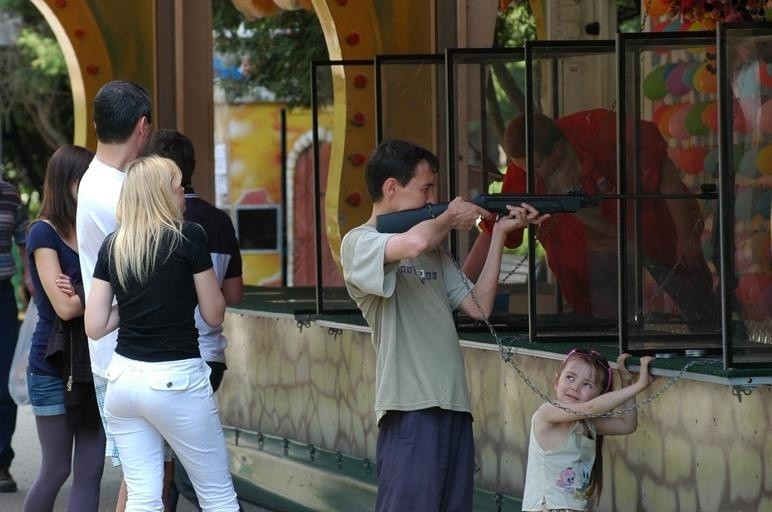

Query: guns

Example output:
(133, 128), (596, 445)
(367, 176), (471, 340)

(376, 183), (720, 233)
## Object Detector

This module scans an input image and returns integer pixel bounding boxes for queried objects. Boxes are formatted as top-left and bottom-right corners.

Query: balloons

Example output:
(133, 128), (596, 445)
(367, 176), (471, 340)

(638, 2), (771, 324)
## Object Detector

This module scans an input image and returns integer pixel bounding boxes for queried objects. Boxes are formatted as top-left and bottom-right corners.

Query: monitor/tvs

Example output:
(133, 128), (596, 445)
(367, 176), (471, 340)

(233, 205), (282, 254)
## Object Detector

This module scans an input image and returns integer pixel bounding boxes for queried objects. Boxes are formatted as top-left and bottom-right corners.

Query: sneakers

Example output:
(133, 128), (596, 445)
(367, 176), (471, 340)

(686, 335), (715, 358)
(588, 317), (618, 331)
(0, 469), (16, 492)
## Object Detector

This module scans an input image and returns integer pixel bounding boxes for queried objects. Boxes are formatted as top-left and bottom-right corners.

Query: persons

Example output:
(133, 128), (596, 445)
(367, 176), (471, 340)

(338, 137), (552, 510)
(520, 345), (659, 511)
(82, 151), (244, 511)
(73, 78), (175, 510)
(21, 143), (106, 510)
(1, 175), (34, 492)
(456, 109), (720, 336)
(149, 128), (244, 511)
